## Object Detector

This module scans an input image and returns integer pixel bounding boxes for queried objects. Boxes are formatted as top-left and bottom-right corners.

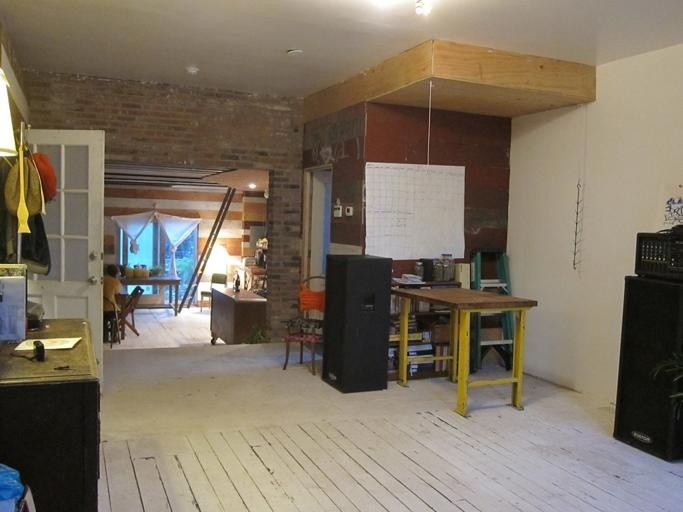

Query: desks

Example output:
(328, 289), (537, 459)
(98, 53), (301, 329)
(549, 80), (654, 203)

(391, 287), (537, 416)
(119, 274), (181, 316)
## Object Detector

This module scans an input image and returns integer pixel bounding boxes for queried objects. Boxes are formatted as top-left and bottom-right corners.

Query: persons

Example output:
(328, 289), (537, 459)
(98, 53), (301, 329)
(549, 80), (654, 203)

(102, 263), (121, 343)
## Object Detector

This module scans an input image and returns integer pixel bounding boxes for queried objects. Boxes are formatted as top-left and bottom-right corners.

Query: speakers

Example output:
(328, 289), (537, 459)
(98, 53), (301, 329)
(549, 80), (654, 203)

(613, 276), (683, 462)
(321, 255), (393, 393)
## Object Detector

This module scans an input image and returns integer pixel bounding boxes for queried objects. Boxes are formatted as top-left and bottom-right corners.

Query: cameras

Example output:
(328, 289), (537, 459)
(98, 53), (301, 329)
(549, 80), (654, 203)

(32, 341), (45, 362)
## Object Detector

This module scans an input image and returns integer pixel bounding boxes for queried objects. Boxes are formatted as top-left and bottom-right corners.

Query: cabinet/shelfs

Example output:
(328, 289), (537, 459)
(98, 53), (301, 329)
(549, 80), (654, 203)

(387, 283), (461, 380)
(471, 251), (515, 373)
(0, 317), (98, 512)
(212, 288), (268, 345)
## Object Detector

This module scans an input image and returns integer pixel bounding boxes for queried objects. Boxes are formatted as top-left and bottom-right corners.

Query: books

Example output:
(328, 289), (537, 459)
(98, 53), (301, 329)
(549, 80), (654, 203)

(388, 273), (452, 378)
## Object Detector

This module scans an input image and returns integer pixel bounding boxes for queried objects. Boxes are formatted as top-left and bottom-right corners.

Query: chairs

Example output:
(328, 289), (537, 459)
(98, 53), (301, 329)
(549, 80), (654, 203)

(282, 276), (328, 378)
(200, 274), (227, 312)
(103, 286), (144, 349)
